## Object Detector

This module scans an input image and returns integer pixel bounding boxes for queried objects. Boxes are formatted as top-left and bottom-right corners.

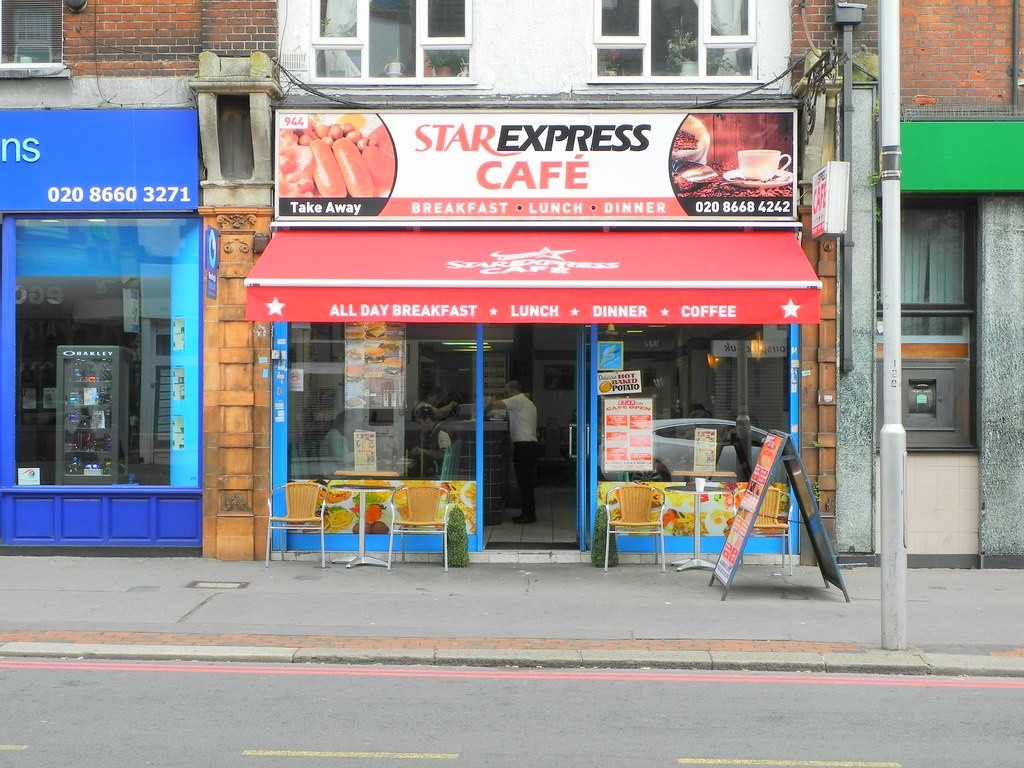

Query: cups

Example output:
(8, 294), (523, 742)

(737, 150), (791, 182)
(695, 477), (705, 492)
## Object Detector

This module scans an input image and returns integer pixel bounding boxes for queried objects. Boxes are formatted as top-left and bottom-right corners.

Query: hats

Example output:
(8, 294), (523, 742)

(414, 403), (435, 417)
(689, 403), (711, 415)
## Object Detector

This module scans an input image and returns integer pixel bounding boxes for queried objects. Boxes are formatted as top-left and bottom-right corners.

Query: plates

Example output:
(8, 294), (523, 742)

(723, 170), (793, 188)
(345, 395), (366, 408)
(490, 418), (505, 421)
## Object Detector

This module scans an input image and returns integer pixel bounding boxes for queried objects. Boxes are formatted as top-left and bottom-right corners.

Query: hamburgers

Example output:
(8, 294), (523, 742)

(345, 322), (405, 382)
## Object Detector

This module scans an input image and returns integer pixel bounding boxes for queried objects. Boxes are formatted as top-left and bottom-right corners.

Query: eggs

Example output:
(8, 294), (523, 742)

(709, 515), (727, 535)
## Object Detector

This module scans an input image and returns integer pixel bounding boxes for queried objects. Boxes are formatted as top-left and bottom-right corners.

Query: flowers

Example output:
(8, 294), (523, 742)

(598, 50), (620, 71)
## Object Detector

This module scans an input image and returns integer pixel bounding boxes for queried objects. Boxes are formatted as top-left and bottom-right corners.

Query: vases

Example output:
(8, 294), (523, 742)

(598, 67), (619, 76)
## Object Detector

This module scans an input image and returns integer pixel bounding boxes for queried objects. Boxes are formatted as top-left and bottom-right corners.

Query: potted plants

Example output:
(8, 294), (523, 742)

(424, 50), (453, 77)
(384, 47), (404, 76)
(666, 30), (741, 76)
(320, 17), (355, 77)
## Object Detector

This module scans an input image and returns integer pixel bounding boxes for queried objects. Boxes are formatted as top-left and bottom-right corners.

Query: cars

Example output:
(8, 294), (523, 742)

(596, 417), (790, 484)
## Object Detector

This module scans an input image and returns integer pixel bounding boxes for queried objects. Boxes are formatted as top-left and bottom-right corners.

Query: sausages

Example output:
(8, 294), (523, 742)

(309, 137), (395, 198)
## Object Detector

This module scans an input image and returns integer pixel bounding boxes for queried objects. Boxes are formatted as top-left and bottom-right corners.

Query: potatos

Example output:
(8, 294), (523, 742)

(353, 521), (389, 534)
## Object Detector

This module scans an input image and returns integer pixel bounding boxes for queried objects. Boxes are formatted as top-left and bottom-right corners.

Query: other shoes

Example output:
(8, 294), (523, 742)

(512, 511), (536, 523)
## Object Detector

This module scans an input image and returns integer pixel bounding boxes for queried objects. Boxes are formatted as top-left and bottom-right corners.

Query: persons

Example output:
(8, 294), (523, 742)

(410, 406), (452, 477)
(411, 384), (457, 421)
(484, 378), (540, 524)
(319, 411), (354, 478)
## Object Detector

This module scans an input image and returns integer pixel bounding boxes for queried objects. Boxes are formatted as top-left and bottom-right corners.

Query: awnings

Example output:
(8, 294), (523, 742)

(243, 229), (823, 326)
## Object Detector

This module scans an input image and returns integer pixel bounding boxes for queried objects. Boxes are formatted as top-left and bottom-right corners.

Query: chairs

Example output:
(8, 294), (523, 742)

(265, 482), (328, 567)
(733, 488), (793, 576)
(387, 485), (450, 572)
(604, 484), (666, 573)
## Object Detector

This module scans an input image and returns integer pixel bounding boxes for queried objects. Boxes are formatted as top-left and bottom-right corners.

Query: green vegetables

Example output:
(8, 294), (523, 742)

(352, 491), (382, 505)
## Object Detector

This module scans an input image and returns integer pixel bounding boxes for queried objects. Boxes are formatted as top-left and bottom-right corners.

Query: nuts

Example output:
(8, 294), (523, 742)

(290, 120), (376, 151)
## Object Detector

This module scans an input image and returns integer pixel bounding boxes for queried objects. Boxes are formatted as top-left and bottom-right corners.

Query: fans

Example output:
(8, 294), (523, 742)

(586, 323), (645, 338)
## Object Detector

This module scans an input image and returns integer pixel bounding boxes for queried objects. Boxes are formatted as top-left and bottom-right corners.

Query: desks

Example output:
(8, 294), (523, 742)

(668, 464), (738, 482)
(329, 484), (396, 568)
(333, 457), (416, 480)
(664, 486), (732, 571)
